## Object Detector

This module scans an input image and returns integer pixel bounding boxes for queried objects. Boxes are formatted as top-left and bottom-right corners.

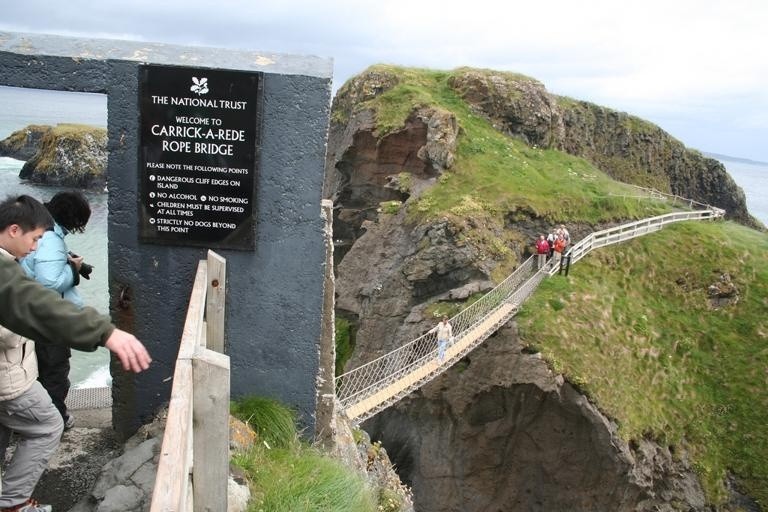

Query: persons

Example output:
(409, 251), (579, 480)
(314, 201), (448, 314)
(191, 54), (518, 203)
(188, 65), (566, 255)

(0, 193), (152, 512)
(17, 190), (91, 432)
(534, 221), (572, 273)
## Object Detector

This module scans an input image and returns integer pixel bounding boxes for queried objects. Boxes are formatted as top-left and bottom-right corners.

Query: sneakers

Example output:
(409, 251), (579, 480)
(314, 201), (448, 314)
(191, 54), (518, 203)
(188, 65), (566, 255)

(63, 412), (76, 432)
(0, 497), (54, 512)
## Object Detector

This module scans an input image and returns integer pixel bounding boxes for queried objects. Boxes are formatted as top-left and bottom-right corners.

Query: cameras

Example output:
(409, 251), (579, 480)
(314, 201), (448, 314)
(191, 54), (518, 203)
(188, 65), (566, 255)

(66, 251), (92, 280)
